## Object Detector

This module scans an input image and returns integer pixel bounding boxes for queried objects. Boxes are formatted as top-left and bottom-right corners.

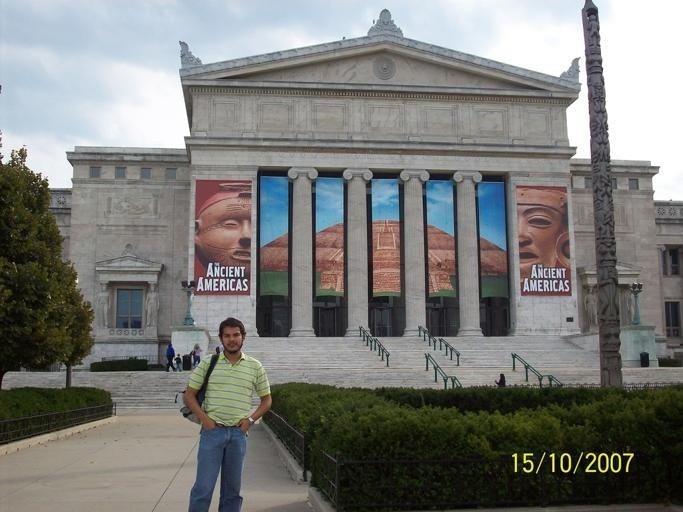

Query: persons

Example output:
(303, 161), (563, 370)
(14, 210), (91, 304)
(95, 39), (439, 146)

(494, 372), (506, 387)
(165, 342), (176, 372)
(191, 186), (250, 278)
(143, 285), (159, 326)
(625, 287), (635, 321)
(178, 316), (274, 512)
(582, 287), (601, 330)
(190, 342), (202, 367)
(174, 354), (181, 372)
(95, 285), (111, 327)
(513, 185), (568, 280)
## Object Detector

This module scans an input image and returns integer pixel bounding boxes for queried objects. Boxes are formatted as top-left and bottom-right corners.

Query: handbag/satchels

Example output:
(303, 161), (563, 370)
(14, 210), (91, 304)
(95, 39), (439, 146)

(177, 391), (201, 424)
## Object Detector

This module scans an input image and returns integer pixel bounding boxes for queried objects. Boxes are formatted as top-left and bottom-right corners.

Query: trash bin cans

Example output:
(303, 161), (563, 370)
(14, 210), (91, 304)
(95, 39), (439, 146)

(183, 355), (191, 370)
(640, 352), (649, 367)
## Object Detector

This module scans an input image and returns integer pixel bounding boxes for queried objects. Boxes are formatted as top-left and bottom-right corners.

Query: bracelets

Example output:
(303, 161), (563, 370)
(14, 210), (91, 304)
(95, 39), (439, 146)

(247, 416), (255, 424)
